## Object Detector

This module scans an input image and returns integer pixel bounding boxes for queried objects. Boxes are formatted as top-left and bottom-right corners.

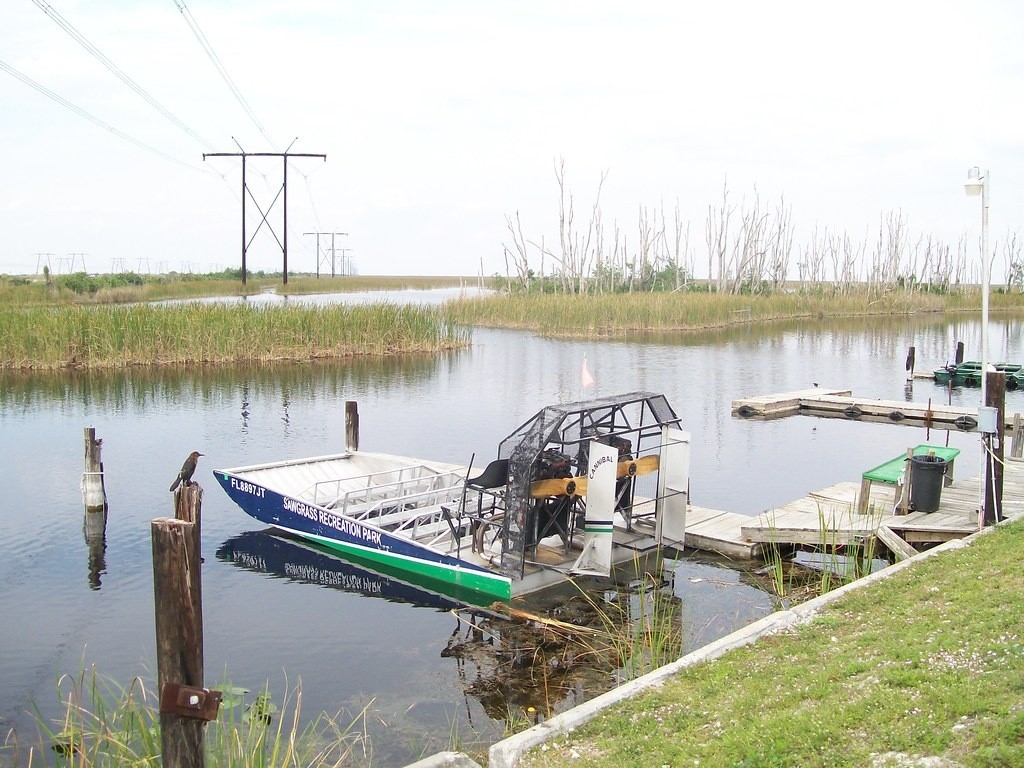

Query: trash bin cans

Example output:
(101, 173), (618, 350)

(910, 455), (949, 513)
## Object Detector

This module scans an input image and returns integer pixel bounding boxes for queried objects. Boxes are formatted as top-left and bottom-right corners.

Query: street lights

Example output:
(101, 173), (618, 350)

(964, 165), (993, 534)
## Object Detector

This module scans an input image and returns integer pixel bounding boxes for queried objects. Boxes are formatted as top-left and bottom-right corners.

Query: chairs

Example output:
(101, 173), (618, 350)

(461, 457), (509, 518)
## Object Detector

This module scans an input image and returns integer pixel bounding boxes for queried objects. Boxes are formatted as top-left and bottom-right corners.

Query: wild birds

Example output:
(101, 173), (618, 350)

(169, 451), (205, 492)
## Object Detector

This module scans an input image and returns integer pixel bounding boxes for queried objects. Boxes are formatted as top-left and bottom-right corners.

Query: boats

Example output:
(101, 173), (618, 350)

(212, 389), (684, 625)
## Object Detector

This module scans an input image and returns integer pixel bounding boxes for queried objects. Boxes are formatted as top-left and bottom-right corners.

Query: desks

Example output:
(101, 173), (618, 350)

(857, 446), (961, 516)
